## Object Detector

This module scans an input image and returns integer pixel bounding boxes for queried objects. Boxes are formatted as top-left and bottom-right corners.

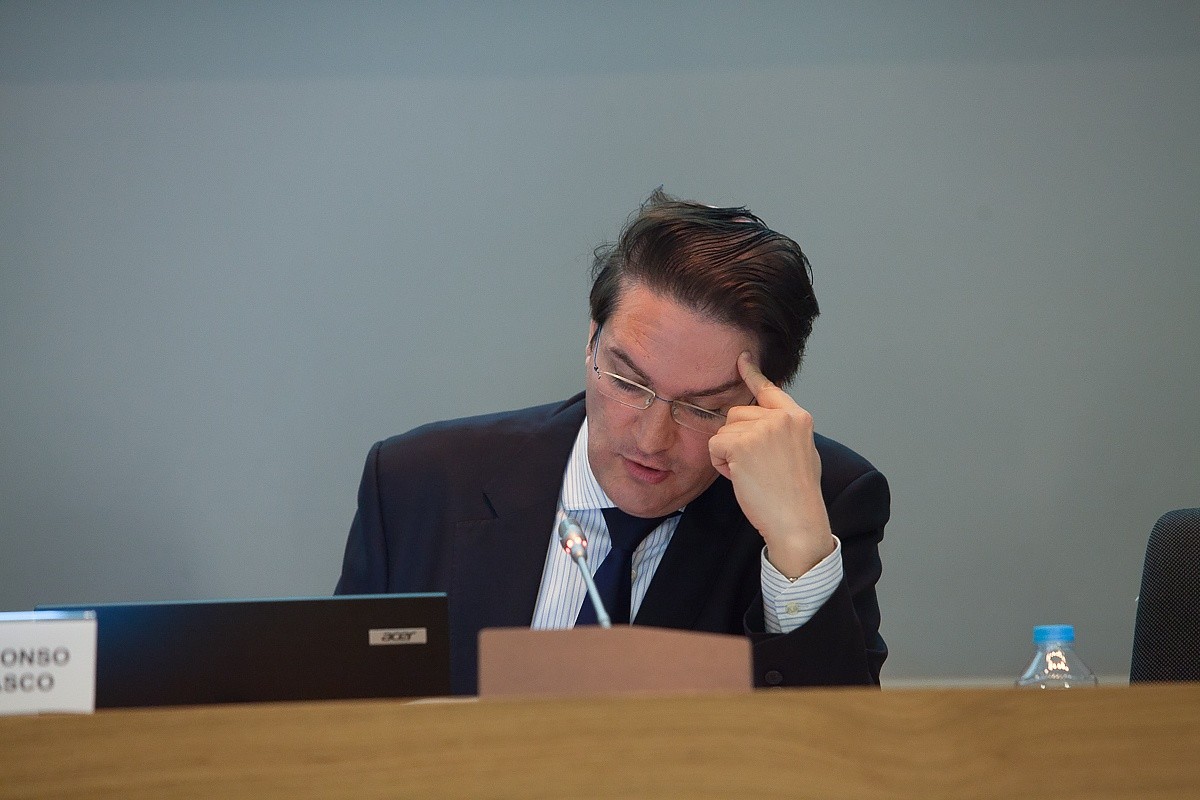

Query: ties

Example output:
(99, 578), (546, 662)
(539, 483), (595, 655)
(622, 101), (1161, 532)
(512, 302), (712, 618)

(575, 507), (682, 624)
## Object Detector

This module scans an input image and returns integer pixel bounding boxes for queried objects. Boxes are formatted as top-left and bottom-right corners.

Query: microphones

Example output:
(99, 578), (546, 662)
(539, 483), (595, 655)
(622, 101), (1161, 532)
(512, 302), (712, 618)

(558, 518), (610, 629)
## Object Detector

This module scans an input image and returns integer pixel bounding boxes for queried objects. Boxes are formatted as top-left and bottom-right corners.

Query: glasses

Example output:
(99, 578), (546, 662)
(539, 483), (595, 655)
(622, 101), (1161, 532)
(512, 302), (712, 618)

(594, 325), (756, 435)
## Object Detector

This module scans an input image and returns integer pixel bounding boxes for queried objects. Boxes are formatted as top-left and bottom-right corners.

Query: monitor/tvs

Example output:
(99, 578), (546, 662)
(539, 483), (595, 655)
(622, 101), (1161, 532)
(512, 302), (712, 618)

(0, 591), (454, 714)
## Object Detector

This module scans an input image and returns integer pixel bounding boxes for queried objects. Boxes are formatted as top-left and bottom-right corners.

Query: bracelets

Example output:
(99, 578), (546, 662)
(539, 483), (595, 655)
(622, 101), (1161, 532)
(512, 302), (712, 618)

(783, 575), (800, 582)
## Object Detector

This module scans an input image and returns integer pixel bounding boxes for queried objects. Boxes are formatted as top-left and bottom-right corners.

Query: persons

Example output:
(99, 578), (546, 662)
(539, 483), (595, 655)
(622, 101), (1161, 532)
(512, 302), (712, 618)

(334, 188), (891, 687)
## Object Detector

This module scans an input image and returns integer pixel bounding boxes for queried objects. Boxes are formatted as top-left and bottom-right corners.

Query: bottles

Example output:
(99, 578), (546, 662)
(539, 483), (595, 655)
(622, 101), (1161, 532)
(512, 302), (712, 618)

(1016, 623), (1097, 688)
